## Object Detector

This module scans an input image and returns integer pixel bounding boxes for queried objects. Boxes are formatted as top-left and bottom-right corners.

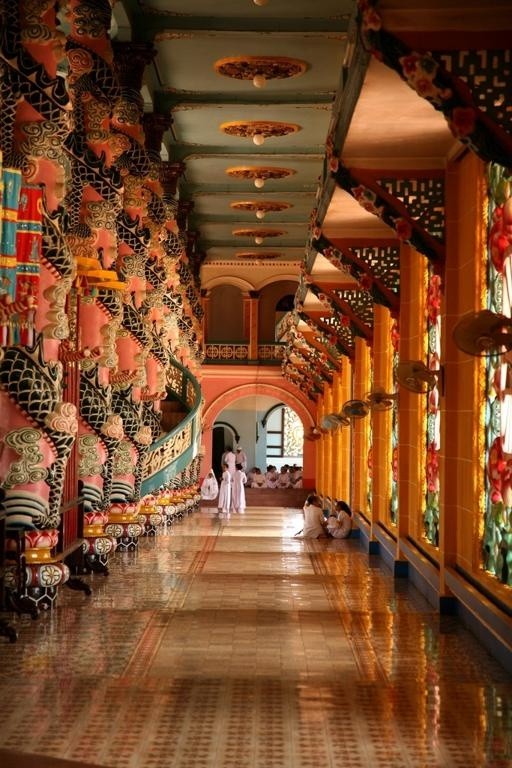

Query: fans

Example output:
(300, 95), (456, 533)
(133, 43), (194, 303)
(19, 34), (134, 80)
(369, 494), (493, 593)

(319, 310), (512, 432)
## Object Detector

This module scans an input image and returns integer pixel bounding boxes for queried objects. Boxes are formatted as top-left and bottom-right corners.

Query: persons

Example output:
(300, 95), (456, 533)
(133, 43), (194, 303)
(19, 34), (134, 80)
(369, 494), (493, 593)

(245, 465), (303, 489)
(221, 447), (236, 468)
(200, 468), (219, 501)
(235, 447), (246, 470)
(231, 464), (248, 514)
(217, 464), (232, 513)
(326, 501), (352, 539)
(303, 493), (329, 540)
